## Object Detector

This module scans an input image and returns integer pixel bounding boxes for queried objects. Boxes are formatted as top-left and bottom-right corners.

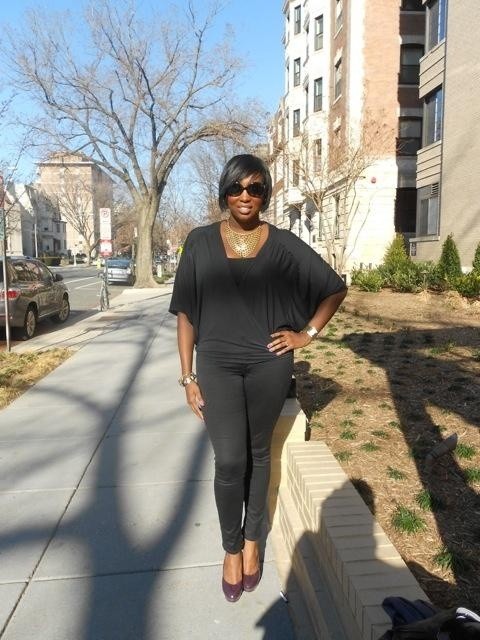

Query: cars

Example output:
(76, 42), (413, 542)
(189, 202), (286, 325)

(104, 253), (177, 285)
(0, 255), (71, 340)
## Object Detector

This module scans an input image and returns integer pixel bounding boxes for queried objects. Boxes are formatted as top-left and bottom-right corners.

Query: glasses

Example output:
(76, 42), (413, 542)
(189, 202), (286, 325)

(226, 182), (263, 197)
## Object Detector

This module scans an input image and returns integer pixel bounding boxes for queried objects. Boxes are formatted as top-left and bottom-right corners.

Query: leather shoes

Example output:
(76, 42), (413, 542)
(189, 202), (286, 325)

(243, 567), (260, 592)
(222, 574), (244, 602)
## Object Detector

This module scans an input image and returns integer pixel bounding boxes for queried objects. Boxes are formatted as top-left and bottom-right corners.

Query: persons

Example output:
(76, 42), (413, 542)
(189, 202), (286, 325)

(166, 153), (348, 603)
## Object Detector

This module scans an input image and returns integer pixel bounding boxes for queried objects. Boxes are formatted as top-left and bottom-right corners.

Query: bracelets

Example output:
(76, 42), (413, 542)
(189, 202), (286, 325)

(177, 371), (196, 387)
(303, 323), (319, 339)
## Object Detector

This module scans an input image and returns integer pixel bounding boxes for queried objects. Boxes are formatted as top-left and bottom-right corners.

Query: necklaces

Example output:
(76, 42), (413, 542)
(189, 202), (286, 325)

(224, 217), (263, 259)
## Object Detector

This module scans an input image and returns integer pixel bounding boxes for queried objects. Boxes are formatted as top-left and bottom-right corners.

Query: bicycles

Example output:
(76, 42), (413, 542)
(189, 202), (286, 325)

(96, 269), (113, 313)
